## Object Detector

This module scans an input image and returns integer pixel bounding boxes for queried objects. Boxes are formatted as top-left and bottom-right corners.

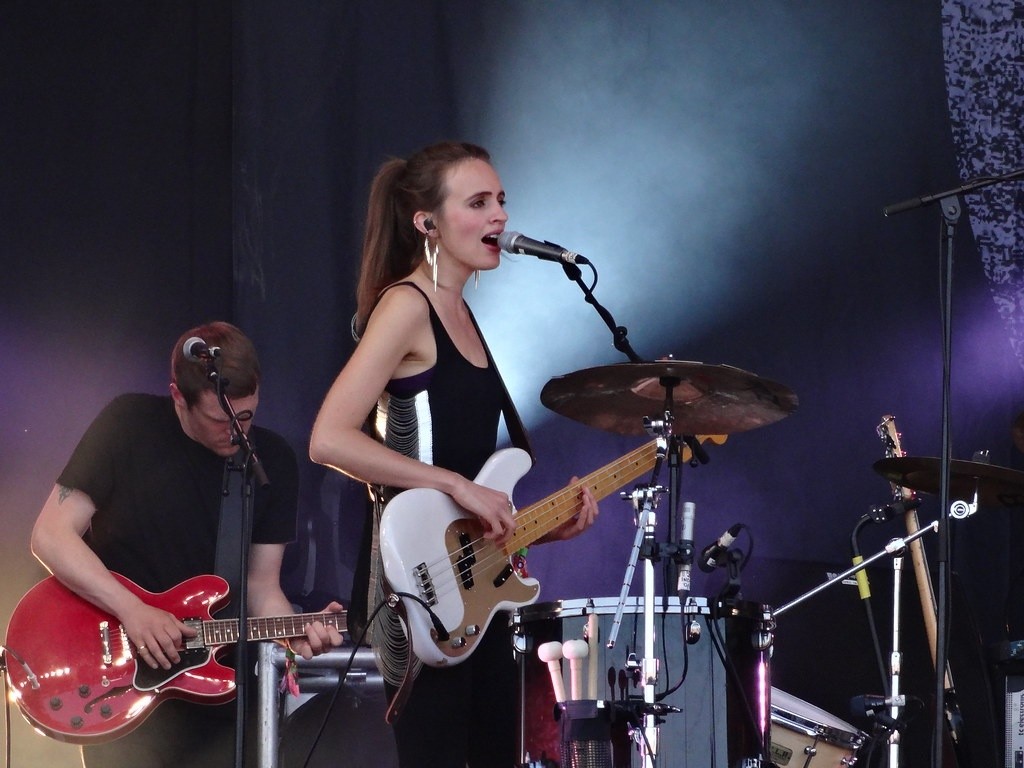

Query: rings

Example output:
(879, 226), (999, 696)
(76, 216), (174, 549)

(136, 645), (149, 654)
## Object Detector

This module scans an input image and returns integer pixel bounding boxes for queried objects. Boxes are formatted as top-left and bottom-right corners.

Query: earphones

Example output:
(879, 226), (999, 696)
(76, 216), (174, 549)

(423, 217), (436, 231)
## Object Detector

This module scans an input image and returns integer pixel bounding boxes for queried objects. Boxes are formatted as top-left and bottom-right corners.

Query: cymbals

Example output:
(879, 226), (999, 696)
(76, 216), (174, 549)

(539, 357), (794, 440)
(875, 453), (1023, 498)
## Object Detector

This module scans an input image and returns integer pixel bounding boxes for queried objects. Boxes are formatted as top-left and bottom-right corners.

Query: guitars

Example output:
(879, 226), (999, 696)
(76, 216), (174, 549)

(382, 435), (729, 668)
(4, 570), (372, 745)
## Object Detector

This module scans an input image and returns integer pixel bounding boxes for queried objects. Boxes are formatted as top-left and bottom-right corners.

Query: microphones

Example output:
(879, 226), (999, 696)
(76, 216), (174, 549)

(677, 501), (696, 606)
(497, 231), (589, 265)
(182, 336), (222, 362)
(697, 523), (743, 573)
(850, 694), (918, 718)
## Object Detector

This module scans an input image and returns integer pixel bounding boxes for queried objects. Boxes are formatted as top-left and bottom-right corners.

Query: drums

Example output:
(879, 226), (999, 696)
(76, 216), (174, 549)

(769, 684), (869, 768)
(506, 590), (773, 768)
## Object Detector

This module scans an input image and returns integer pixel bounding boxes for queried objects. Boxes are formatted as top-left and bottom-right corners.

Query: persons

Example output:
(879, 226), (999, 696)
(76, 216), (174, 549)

(30, 319), (343, 768)
(306, 138), (600, 768)
